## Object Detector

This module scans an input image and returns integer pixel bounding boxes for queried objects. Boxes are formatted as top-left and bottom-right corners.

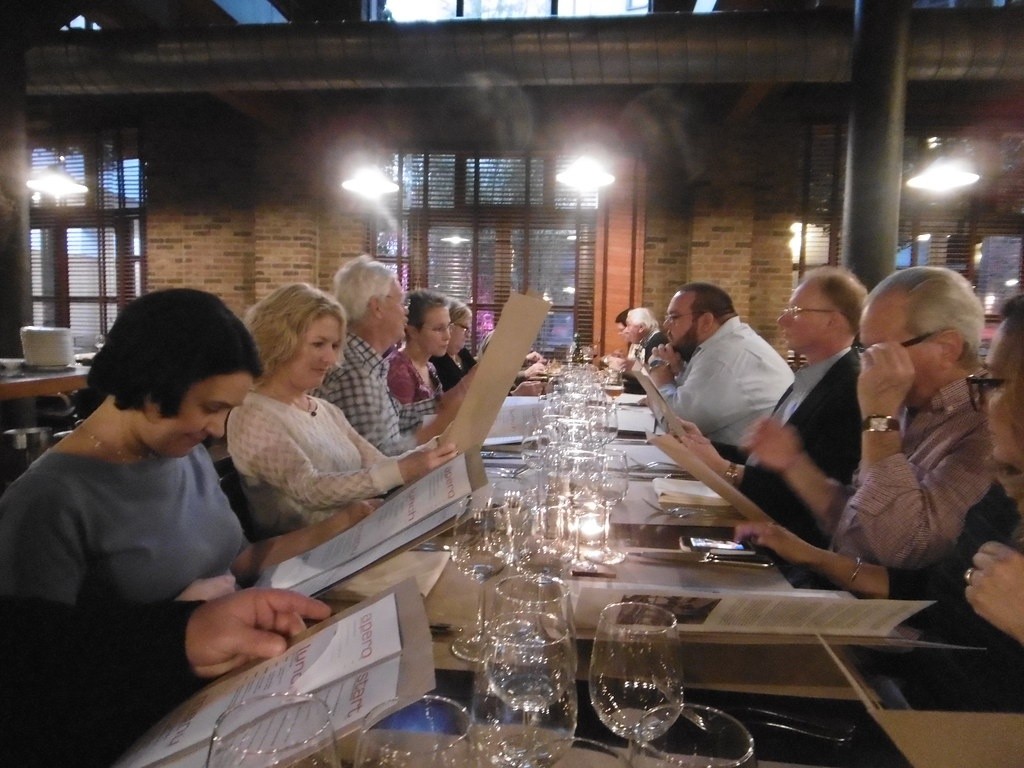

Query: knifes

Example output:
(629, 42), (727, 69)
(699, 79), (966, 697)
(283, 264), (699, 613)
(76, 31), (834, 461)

(626, 550), (774, 569)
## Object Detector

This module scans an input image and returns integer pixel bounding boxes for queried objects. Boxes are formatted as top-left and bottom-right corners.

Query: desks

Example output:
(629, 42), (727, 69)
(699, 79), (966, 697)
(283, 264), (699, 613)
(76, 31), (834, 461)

(279, 365), (1024, 768)
(0, 359), (95, 493)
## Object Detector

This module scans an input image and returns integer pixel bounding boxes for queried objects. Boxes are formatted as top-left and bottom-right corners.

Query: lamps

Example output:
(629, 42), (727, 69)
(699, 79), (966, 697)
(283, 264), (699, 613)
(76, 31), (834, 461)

(24, 159), (91, 201)
(904, 149), (978, 196)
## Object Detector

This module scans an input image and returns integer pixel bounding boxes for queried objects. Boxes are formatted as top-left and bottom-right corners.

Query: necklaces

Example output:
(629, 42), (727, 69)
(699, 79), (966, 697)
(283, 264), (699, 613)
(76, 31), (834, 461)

(454, 356), (461, 367)
(80, 427), (143, 463)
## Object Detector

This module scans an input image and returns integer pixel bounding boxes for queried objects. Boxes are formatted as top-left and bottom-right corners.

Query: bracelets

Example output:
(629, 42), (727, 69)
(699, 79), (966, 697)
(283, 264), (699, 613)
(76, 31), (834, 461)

(850, 557), (862, 580)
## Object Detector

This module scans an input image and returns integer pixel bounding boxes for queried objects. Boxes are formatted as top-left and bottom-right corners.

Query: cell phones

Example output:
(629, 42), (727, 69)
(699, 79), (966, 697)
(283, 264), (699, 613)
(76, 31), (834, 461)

(678, 535), (756, 555)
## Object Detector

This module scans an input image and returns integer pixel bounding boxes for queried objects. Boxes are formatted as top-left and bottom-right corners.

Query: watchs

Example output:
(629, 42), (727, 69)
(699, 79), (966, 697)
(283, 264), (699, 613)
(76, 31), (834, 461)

(862, 415), (899, 432)
(725, 462), (738, 479)
(649, 360), (670, 372)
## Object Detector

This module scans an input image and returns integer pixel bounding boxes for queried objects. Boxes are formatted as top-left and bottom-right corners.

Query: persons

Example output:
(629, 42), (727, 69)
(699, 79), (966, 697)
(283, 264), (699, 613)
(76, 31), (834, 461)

(429, 300), (547, 402)
(619, 307), (668, 394)
(303, 252), (481, 457)
(668, 265), (869, 569)
(742, 265), (995, 597)
(613, 305), (635, 359)
(736, 293), (1024, 714)
(0, 288), (372, 768)
(476, 330), (541, 366)
(647, 281), (795, 464)
(387, 288), (455, 405)
(226, 282), (458, 531)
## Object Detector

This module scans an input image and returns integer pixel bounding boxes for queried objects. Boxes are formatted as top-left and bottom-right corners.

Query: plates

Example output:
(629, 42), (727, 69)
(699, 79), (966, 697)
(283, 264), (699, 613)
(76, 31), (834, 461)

(19, 325), (76, 372)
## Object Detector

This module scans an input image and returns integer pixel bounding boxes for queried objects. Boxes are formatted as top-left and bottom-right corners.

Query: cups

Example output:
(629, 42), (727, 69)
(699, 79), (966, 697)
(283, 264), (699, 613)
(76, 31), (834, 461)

(353, 693), (472, 768)
(206, 691), (341, 768)
(627, 703), (759, 768)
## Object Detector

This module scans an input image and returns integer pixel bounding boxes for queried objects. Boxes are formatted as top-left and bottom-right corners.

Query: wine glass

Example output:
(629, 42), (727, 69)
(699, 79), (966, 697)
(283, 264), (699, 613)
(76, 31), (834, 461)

(451, 354), (683, 768)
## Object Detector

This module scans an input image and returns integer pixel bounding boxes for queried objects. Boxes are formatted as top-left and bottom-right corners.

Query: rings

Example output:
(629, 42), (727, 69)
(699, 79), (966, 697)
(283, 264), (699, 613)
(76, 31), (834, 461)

(768, 522), (774, 526)
(965, 568), (975, 581)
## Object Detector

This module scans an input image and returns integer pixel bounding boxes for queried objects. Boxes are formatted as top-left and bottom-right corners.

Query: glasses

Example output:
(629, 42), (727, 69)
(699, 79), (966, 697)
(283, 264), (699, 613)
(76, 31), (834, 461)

(663, 311), (705, 326)
(784, 304), (850, 321)
(966, 367), (1007, 414)
(851, 332), (934, 360)
(455, 323), (472, 338)
(386, 294), (411, 310)
(421, 322), (455, 338)
